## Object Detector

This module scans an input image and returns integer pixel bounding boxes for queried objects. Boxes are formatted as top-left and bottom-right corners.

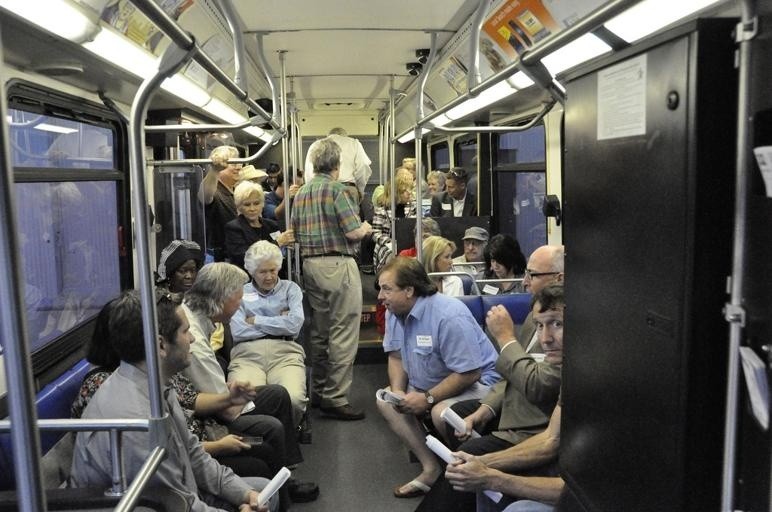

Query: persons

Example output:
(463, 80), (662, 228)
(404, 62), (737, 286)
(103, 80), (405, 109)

(72, 145), (319, 510)
(372, 157), (564, 512)
(17, 146), (119, 353)
(291, 126), (374, 421)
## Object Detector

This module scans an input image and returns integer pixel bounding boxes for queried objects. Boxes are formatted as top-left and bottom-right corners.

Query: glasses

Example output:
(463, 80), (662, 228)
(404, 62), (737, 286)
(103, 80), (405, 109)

(157, 291), (172, 305)
(524, 268), (559, 279)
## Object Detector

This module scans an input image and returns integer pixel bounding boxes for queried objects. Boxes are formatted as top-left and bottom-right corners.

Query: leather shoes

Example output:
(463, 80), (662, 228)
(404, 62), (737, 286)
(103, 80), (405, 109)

(287, 477), (319, 503)
(319, 404), (365, 420)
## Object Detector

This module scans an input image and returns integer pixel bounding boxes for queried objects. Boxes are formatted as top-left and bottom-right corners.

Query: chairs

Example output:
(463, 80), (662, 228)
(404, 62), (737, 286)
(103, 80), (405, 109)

(453, 293), (482, 338)
(480, 288), (531, 350)
(459, 270), (477, 295)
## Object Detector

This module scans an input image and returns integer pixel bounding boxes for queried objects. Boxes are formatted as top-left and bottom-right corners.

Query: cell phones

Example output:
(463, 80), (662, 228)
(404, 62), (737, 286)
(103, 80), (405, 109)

(240, 437), (263, 446)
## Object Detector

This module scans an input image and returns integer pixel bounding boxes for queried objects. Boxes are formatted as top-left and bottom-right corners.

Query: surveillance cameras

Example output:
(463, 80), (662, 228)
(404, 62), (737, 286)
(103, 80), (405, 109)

(416, 49), (430, 64)
(406, 62), (423, 77)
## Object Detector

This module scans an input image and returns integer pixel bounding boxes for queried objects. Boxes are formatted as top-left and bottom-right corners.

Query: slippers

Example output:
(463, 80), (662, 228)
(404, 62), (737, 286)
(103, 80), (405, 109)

(394, 480), (431, 497)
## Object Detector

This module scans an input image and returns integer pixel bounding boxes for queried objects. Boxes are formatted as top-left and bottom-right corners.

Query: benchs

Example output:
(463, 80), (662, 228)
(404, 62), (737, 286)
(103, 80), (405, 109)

(0, 351), (92, 495)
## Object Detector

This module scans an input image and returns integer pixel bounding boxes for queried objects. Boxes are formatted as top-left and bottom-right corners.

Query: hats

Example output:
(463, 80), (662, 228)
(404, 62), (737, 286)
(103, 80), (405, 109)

(461, 226), (490, 241)
(233, 165), (268, 188)
(156, 239), (206, 283)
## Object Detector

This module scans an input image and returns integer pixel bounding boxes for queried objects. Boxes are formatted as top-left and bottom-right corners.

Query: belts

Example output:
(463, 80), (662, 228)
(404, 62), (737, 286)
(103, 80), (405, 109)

(304, 252), (353, 258)
(341, 182), (355, 186)
(261, 334), (294, 341)
